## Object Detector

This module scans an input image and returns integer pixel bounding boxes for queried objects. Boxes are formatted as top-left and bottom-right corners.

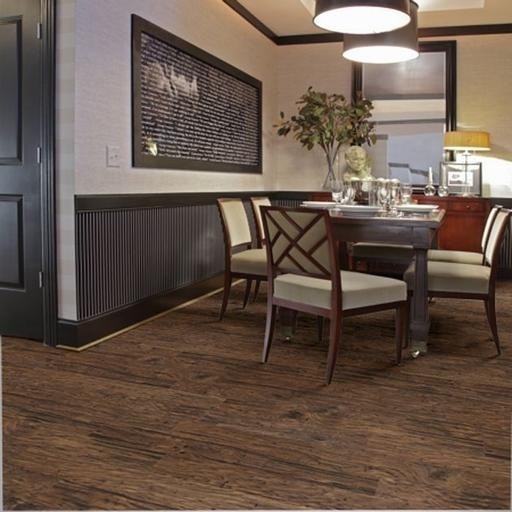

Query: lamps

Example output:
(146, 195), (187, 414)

(443, 131), (490, 196)
(313, 0), (411, 35)
(342, 0), (419, 64)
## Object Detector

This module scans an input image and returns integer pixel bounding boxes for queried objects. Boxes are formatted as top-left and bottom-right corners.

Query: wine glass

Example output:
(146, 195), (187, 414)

(332, 181), (357, 205)
(367, 178), (413, 216)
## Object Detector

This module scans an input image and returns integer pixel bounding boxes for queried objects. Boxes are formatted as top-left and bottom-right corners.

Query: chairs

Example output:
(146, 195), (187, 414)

(214, 196), (274, 321)
(257, 203), (408, 384)
(403, 203), (511, 356)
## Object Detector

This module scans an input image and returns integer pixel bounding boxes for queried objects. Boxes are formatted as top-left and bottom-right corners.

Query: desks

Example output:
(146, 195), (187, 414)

(299, 202), (446, 355)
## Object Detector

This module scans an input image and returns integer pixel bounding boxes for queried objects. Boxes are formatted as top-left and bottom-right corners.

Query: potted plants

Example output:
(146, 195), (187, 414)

(273, 86), (377, 191)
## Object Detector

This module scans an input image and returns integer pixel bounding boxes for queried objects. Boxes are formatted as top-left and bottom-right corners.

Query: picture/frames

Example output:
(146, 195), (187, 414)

(440, 162), (482, 196)
(131, 13), (262, 174)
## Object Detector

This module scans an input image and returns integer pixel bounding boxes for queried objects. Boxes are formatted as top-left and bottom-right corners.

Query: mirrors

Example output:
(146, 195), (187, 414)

(351, 40), (456, 194)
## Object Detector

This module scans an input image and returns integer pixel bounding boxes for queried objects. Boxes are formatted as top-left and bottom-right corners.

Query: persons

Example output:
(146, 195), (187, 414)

(341, 145), (375, 182)
